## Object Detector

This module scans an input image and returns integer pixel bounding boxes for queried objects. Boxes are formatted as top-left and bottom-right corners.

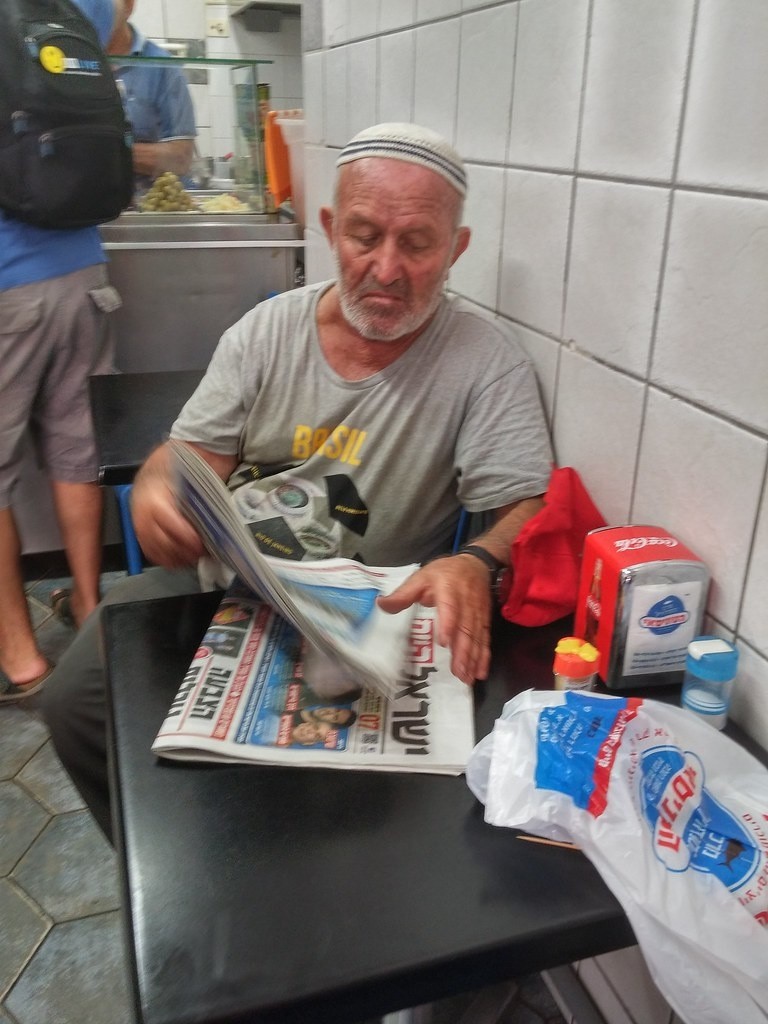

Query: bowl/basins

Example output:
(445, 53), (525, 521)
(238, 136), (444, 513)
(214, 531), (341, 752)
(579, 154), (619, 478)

(208, 179), (236, 190)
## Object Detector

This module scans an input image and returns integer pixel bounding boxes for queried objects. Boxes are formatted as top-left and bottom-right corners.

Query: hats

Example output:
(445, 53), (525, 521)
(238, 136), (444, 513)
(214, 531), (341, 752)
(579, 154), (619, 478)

(336, 121), (468, 198)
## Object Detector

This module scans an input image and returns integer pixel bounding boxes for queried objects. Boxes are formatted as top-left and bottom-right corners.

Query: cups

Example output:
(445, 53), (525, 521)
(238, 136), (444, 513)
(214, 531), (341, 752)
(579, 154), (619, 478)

(272, 118), (307, 226)
(213, 162), (232, 180)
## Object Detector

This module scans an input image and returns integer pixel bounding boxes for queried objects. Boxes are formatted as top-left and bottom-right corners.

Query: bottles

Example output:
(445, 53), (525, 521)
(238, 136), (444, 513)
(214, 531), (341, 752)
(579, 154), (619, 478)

(679, 635), (739, 732)
(552, 636), (599, 693)
(217, 152), (233, 163)
(113, 78), (136, 211)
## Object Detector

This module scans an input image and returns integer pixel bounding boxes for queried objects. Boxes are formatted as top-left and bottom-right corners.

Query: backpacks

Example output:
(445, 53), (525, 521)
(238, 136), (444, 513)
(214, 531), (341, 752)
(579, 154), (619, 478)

(0, 0), (133, 230)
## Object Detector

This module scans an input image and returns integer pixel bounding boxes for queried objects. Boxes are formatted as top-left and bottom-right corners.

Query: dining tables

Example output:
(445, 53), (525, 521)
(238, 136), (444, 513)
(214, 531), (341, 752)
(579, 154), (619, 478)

(92, 588), (768, 1024)
(86, 369), (229, 488)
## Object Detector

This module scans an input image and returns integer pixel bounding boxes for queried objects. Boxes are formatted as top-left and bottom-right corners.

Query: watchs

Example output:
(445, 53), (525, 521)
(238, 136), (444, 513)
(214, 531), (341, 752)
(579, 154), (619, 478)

(459, 542), (512, 605)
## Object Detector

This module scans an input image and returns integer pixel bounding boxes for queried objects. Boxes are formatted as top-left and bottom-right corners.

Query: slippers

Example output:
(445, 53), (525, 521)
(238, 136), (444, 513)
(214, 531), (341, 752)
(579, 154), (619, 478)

(0, 657), (56, 701)
(49, 588), (75, 629)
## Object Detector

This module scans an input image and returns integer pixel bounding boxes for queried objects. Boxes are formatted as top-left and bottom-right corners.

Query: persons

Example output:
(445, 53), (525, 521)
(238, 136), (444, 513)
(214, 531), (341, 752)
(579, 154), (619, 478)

(109, 0), (200, 205)
(0, 0), (136, 699)
(37, 121), (558, 1024)
(286, 684), (366, 746)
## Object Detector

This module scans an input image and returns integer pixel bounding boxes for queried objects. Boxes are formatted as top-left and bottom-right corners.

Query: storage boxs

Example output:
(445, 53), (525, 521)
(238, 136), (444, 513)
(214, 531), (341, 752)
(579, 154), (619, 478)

(573, 524), (710, 696)
